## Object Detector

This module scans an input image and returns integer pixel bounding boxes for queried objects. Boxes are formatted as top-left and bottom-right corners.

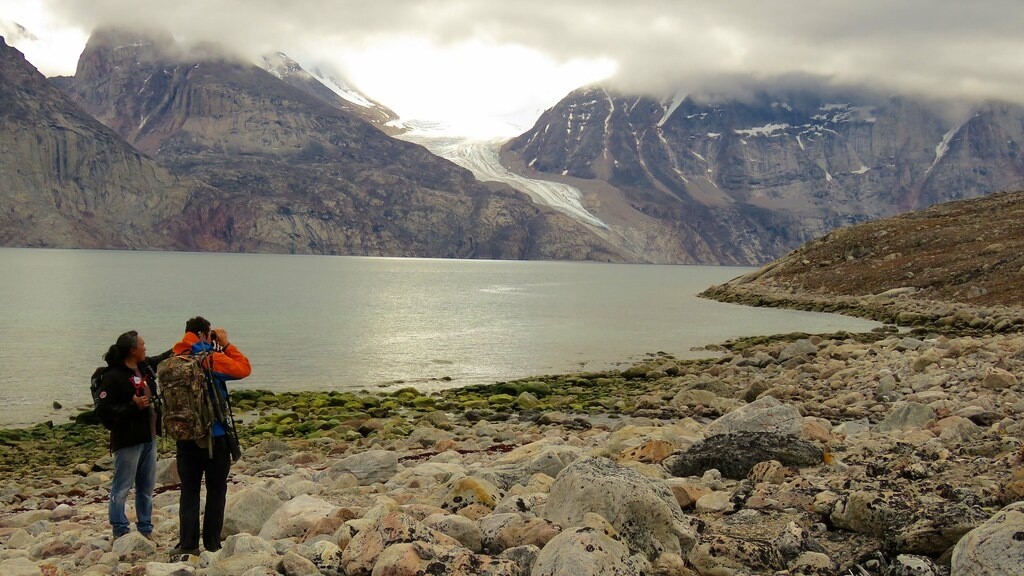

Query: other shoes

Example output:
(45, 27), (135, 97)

(142, 533), (160, 547)
(169, 545), (200, 558)
(110, 537), (119, 546)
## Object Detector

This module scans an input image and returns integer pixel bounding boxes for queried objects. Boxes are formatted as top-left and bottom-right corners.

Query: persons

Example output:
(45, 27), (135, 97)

(106, 331), (174, 546)
(169, 316), (251, 557)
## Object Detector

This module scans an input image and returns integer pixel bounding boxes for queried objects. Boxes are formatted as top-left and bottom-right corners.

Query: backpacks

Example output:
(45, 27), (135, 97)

(90, 360), (158, 430)
(157, 350), (216, 440)
